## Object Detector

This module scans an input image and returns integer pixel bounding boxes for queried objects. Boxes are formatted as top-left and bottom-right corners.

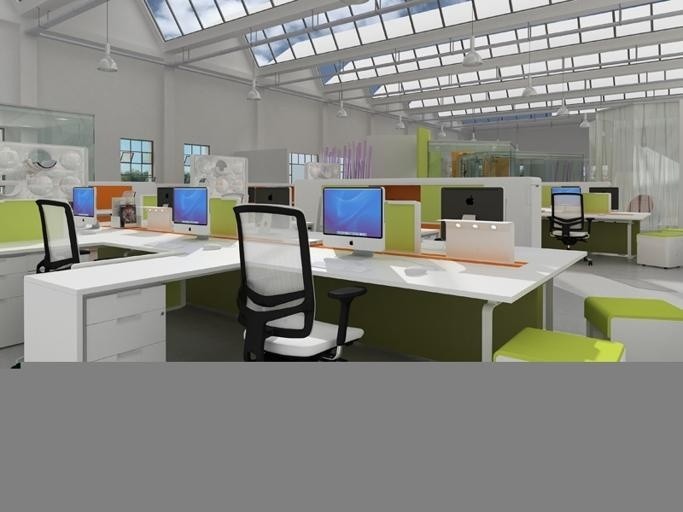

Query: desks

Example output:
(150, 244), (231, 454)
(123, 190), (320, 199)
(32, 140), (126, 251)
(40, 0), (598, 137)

(541, 208), (652, 261)
(0, 226), (589, 361)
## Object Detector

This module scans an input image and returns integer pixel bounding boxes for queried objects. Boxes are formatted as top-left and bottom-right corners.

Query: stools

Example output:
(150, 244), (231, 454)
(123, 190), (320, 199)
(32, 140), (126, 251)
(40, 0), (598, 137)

(583, 297), (683, 362)
(493, 326), (627, 363)
(636, 228), (683, 269)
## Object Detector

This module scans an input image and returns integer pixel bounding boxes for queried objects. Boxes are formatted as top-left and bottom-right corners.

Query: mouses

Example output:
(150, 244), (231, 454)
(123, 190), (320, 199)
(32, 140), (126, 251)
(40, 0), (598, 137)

(202, 242), (221, 250)
(405, 266), (427, 275)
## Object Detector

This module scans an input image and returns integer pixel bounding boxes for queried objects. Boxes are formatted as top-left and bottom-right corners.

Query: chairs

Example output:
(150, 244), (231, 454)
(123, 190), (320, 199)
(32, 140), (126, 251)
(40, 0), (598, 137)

(546, 193), (596, 266)
(11, 199), (110, 368)
(232, 203), (367, 361)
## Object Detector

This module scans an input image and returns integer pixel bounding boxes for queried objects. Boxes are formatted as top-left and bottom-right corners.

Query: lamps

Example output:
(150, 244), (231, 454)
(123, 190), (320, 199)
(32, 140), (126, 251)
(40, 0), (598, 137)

(244, 28), (263, 103)
(96, 0), (120, 71)
(335, 0), (590, 153)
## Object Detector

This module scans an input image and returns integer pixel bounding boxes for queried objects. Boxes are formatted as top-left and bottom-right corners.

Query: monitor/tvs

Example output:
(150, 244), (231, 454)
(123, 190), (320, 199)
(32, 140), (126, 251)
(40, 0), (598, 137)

(157, 187), (174, 207)
(254, 187), (292, 206)
(551, 187), (581, 194)
(73, 186), (100, 232)
(589, 187), (619, 210)
(172, 187), (211, 240)
(440, 187), (506, 241)
(322, 186), (385, 261)
